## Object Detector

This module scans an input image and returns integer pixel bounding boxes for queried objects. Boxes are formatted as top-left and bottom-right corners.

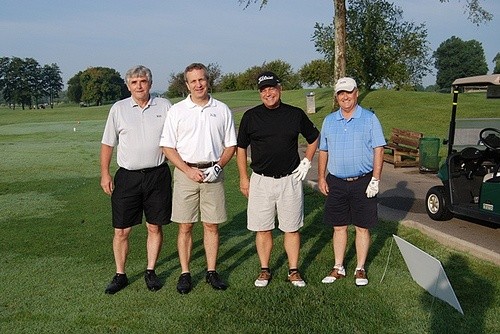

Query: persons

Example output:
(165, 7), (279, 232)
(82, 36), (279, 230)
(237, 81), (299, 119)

(236, 72), (319, 287)
(159, 62), (238, 295)
(100, 64), (173, 294)
(317, 77), (387, 286)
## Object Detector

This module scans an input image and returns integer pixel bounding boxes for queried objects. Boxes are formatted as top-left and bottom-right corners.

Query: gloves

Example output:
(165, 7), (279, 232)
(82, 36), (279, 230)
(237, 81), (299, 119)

(292, 157), (312, 182)
(365, 176), (381, 198)
(202, 164), (223, 182)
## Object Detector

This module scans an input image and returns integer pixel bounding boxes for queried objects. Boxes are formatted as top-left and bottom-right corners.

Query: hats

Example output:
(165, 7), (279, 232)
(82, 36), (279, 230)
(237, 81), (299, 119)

(257, 71), (280, 89)
(333, 77), (357, 94)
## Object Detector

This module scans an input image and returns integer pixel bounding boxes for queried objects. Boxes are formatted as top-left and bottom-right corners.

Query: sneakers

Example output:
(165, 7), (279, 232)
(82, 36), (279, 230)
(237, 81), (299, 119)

(144, 269), (162, 292)
(205, 272), (228, 291)
(176, 273), (192, 295)
(287, 271), (306, 288)
(353, 269), (368, 286)
(104, 273), (129, 295)
(321, 268), (346, 284)
(254, 269), (272, 287)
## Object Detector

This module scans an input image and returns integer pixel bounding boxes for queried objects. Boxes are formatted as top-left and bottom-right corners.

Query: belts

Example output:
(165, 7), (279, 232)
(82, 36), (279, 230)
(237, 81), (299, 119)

(342, 170), (374, 182)
(183, 160), (219, 169)
(124, 163), (166, 174)
(252, 169), (293, 179)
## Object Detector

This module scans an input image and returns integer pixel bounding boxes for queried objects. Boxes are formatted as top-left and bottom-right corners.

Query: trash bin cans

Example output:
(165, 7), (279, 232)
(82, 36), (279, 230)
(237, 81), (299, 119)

(416, 137), (442, 175)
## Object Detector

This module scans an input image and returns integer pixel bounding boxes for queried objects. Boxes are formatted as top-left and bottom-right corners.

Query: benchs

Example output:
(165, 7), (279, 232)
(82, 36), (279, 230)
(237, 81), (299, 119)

(385, 128), (422, 167)
